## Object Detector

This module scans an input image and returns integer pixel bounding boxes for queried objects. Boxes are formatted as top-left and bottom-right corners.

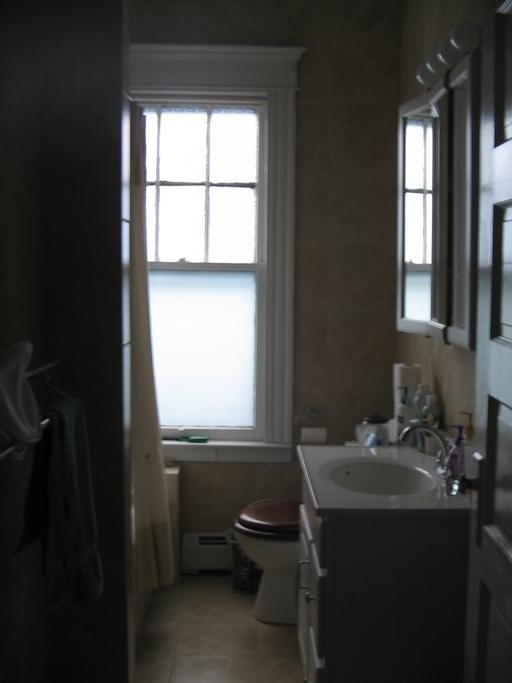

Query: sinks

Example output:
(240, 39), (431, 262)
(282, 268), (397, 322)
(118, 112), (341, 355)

(323, 457), (435, 497)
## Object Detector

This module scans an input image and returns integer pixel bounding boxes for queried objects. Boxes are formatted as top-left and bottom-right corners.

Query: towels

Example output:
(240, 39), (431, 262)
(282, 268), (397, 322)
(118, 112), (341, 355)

(2, 339), (45, 446)
(43, 398), (103, 604)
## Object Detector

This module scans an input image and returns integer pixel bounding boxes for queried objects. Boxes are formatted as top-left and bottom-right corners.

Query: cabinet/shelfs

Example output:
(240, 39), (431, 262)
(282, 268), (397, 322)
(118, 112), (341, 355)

(293, 504), (475, 683)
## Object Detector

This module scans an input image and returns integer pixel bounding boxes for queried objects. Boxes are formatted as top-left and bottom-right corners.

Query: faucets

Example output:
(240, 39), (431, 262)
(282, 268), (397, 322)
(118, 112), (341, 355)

(400, 426), (450, 496)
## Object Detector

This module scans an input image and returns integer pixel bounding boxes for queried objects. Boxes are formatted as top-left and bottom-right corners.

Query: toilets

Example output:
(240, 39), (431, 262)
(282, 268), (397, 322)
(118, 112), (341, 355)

(234, 501), (301, 625)
